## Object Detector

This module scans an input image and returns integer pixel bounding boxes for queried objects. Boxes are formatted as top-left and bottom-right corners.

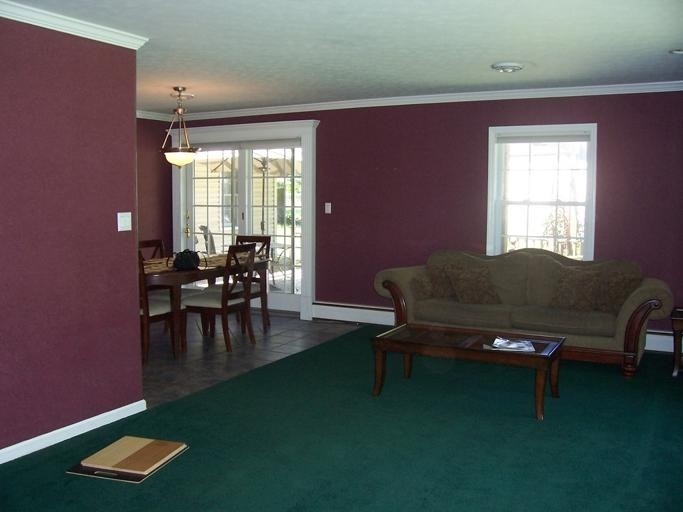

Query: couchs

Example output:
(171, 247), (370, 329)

(374, 248), (674, 381)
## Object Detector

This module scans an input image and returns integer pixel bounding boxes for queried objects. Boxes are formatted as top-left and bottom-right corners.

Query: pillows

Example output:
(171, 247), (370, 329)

(549, 267), (600, 312)
(444, 264), (503, 305)
(428, 263), (464, 298)
(594, 271), (643, 316)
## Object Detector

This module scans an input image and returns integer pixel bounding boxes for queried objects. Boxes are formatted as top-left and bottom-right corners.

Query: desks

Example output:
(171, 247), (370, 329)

(144, 250), (268, 361)
(371, 323), (566, 421)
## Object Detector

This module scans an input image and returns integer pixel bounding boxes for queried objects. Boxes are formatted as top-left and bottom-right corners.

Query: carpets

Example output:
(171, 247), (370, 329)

(0, 324), (683, 512)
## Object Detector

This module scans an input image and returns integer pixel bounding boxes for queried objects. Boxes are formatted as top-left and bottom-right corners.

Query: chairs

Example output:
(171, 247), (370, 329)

(138, 238), (204, 335)
(138, 250), (190, 366)
(182, 243), (256, 353)
(224, 236), (273, 335)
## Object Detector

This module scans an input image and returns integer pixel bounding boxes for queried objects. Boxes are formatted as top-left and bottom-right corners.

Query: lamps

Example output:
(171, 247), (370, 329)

(159, 86), (203, 168)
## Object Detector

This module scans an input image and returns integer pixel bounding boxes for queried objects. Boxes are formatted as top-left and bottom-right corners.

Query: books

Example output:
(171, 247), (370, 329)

(490, 336), (536, 353)
